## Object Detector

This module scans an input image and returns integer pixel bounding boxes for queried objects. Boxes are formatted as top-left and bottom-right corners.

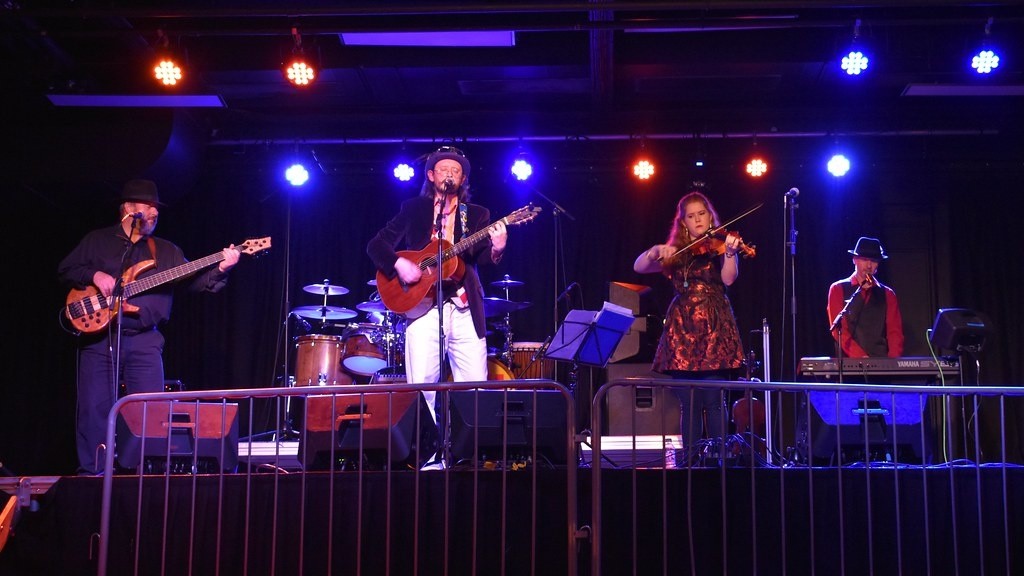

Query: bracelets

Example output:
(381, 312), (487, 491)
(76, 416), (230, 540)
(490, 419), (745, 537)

(726, 252), (734, 258)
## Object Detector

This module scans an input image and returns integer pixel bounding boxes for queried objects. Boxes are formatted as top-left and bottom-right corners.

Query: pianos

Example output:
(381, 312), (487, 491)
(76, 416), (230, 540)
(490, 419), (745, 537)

(796, 355), (960, 468)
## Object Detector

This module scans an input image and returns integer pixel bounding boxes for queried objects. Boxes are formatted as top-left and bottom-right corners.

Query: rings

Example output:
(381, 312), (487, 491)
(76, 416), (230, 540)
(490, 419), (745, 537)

(227, 253), (230, 255)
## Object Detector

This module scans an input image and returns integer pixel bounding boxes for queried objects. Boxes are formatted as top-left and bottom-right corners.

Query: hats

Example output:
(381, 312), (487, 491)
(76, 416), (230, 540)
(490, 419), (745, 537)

(115, 180), (168, 210)
(425, 147), (470, 186)
(847, 236), (889, 260)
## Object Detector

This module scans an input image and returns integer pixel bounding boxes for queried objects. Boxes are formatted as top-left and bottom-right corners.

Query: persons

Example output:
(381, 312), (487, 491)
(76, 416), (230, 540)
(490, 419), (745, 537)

(633, 193), (746, 462)
(58, 180), (240, 475)
(827, 237), (903, 357)
(363, 148), (507, 469)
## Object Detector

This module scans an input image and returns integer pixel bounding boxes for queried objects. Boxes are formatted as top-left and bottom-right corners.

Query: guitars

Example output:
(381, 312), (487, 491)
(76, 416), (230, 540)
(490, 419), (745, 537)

(65, 236), (272, 335)
(376, 201), (543, 319)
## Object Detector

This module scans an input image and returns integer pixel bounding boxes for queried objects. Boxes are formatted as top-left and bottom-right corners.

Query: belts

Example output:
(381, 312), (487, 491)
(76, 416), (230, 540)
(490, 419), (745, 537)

(111, 327), (142, 336)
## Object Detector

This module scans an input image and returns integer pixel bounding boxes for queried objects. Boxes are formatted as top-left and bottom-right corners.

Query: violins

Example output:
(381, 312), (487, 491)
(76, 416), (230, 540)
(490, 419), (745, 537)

(690, 227), (756, 259)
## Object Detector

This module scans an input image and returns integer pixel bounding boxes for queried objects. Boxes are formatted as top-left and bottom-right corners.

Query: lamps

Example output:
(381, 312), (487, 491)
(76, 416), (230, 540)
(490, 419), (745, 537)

(285, 25), (319, 88)
(970, 17), (1000, 74)
(153, 34), (185, 86)
(839, 17), (869, 75)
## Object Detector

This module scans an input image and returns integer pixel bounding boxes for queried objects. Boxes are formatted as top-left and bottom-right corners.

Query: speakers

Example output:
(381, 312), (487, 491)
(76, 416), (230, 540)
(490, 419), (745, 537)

(117, 401), (240, 474)
(446, 391), (577, 467)
(297, 390), (442, 470)
(795, 393), (934, 466)
(603, 363), (682, 437)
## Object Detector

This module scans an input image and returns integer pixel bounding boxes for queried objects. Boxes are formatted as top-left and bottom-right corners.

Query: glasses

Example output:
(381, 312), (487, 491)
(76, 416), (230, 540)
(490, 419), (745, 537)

(433, 168), (463, 178)
(437, 146), (465, 157)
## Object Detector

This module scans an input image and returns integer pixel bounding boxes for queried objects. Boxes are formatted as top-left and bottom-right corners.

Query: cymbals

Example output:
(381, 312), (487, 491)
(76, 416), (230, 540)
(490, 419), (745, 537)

(356, 301), (389, 311)
(484, 297), (534, 311)
(367, 280), (376, 286)
(292, 305), (358, 320)
(302, 284), (349, 295)
(489, 280), (524, 286)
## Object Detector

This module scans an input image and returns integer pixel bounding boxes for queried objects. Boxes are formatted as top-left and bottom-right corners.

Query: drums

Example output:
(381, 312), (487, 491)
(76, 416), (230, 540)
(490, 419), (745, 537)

(507, 341), (555, 379)
(294, 322), (407, 386)
(487, 356), (515, 379)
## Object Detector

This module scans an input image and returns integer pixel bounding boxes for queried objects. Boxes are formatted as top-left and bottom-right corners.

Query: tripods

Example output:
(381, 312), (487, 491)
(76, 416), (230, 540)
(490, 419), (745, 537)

(241, 183), (300, 442)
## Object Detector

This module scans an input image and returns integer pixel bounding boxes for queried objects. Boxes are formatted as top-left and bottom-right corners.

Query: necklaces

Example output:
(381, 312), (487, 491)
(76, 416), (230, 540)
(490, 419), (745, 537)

(683, 253), (696, 287)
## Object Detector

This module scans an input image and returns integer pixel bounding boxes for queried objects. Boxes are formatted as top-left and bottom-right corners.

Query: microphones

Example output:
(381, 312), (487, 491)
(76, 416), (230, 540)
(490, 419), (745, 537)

(784, 188), (800, 198)
(444, 178), (455, 186)
(865, 272), (874, 285)
(129, 210), (146, 218)
(557, 283), (577, 302)
